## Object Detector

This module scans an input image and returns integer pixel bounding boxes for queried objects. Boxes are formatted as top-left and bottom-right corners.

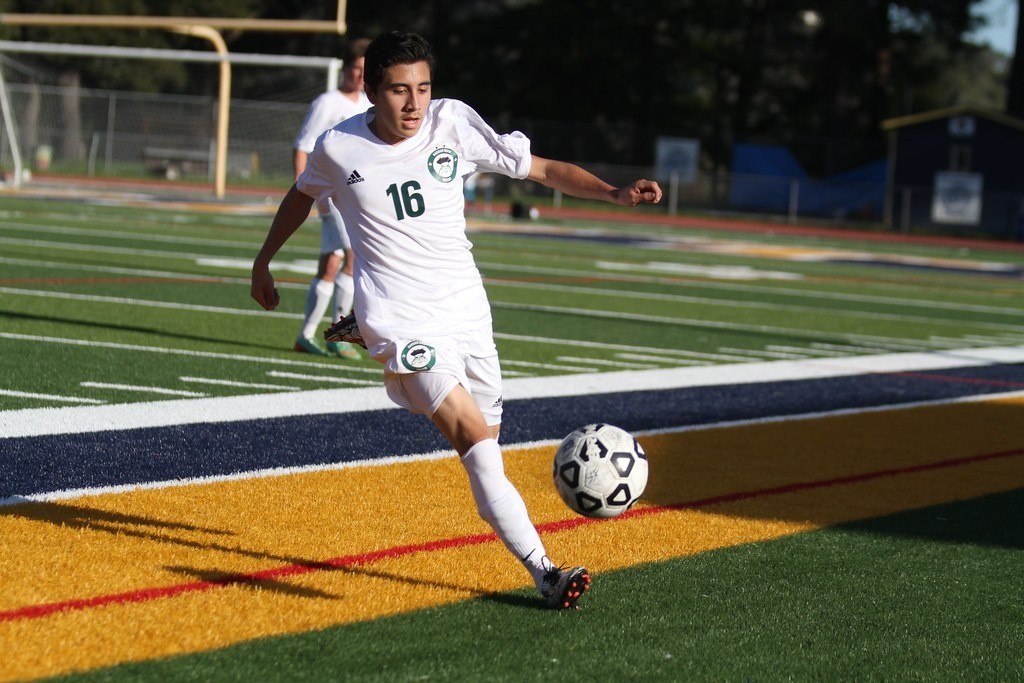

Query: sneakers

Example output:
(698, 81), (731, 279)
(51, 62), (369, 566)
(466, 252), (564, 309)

(323, 309), (369, 350)
(296, 334), (331, 357)
(326, 340), (362, 360)
(541, 555), (590, 608)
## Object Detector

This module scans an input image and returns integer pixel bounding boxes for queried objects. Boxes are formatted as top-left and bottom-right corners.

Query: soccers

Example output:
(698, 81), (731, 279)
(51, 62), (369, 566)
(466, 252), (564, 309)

(552, 422), (649, 518)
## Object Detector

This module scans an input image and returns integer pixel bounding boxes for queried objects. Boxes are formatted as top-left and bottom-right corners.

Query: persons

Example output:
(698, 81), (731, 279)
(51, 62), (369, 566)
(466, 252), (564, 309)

(249, 28), (663, 611)
(292, 38), (371, 359)
